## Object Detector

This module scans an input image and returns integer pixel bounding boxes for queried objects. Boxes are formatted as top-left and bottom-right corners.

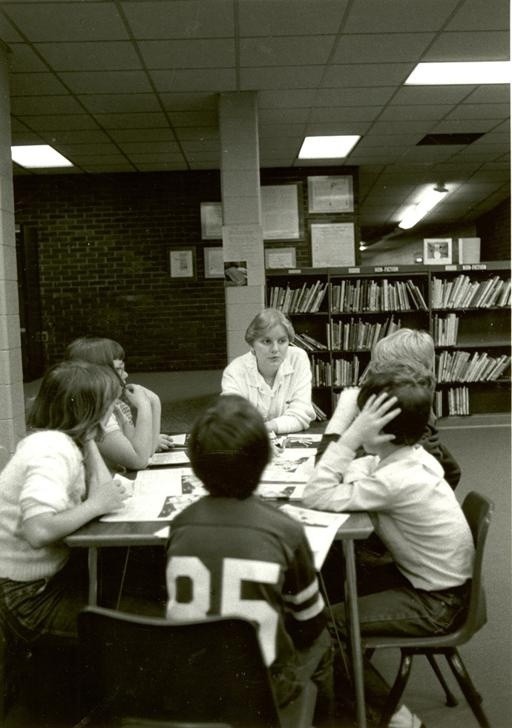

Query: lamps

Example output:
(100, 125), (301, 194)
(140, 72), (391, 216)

(397, 184), (449, 230)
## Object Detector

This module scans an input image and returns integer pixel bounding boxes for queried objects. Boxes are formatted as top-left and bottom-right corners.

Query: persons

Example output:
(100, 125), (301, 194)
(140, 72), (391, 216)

(298, 370), (477, 728)
(313, 324), (461, 491)
(162, 390), (339, 727)
(60, 334), (162, 472)
(217, 308), (313, 435)
(2, 359), (169, 656)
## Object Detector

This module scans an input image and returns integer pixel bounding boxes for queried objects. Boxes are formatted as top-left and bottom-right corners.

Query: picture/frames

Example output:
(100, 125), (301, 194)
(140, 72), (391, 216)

(166, 174), (357, 283)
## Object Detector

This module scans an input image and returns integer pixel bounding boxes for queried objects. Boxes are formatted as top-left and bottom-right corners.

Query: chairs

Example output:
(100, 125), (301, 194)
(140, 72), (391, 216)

(361, 490), (490, 728)
(76, 605), (319, 728)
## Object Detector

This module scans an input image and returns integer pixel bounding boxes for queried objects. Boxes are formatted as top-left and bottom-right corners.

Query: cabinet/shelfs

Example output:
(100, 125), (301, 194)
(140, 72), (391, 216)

(266, 260), (511, 429)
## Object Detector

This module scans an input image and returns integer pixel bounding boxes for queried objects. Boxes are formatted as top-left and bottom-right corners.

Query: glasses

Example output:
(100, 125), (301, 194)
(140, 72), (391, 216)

(115, 362), (124, 377)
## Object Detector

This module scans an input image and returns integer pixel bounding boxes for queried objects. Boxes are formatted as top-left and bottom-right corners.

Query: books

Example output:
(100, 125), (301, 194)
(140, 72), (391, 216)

(265, 267), (510, 415)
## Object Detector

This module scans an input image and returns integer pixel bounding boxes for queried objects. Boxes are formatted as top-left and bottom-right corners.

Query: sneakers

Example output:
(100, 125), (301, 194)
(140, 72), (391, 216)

(388, 704), (426, 727)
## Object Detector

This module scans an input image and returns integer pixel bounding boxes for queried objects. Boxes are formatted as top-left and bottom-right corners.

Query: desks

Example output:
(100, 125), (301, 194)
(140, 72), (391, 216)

(64, 427), (375, 728)
